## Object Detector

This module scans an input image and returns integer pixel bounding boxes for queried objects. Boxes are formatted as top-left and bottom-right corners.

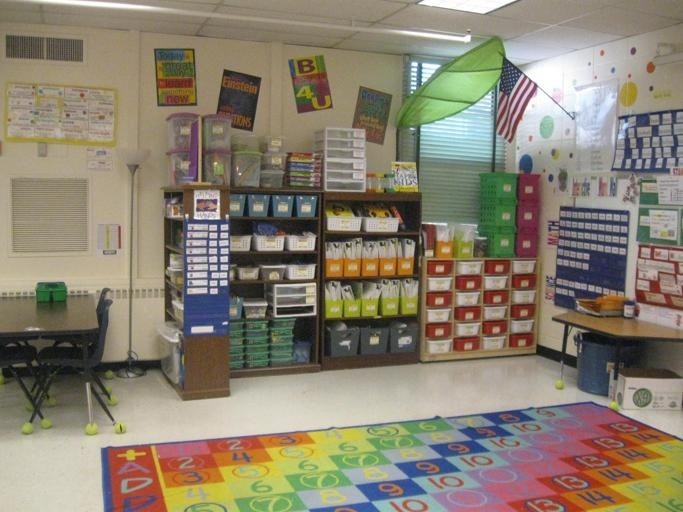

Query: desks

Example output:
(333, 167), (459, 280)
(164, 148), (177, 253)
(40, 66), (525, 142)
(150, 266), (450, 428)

(551, 310), (682, 391)
(0, 295), (101, 434)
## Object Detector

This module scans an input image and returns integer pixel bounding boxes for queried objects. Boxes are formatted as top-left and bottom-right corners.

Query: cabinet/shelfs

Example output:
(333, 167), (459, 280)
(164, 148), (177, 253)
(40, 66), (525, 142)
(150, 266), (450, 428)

(320, 192), (423, 372)
(228, 188), (322, 378)
(163, 183), (228, 400)
(422, 257), (538, 364)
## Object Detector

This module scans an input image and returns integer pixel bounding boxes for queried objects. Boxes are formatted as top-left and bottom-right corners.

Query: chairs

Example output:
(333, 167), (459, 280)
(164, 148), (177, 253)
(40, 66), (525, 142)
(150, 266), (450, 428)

(0, 338), (57, 428)
(22, 298), (123, 438)
(19, 286), (119, 410)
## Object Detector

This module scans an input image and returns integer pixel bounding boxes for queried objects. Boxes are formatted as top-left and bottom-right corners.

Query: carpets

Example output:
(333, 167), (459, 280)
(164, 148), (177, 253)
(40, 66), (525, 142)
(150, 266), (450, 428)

(100, 400), (683, 512)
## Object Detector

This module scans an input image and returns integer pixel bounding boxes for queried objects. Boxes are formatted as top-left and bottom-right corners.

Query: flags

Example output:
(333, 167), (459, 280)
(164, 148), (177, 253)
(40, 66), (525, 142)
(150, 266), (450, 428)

(493, 54), (541, 144)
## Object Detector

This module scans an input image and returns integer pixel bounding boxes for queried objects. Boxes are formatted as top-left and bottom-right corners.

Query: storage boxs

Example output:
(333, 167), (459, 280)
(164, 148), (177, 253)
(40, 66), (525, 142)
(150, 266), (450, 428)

(519, 172), (540, 200)
(230, 195), (248, 216)
(455, 292), (481, 306)
(230, 234), (253, 251)
(455, 238), (475, 259)
(270, 347), (293, 353)
(381, 258), (396, 277)
(391, 328), (419, 355)
(479, 227), (518, 259)
(248, 353), (270, 360)
(517, 201), (539, 229)
(478, 172), (518, 202)
(426, 309), (451, 323)
(484, 306), (507, 320)
(234, 151), (263, 188)
(260, 264), (285, 281)
(427, 278), (452, 292)
(294, 342), (312, 365)
(229, 361), (245, 370)
(361, 299), (379, 317)
(361, 328), (390, 356)
(485, 261), (509, 274)
(269, 329), (293, 336)
(228, 321), (245, 330)
(228, 330), (244, 338)
(247, 329), (268, 338)
(272, 194), (294, 218)
(484, 321), (506, 334)
(202, 153), (233, 187)
(456, 323), (480, 337)
(455, 307), (481, 321)
(361, 216), (400, 232)
(248, 338), (269, 346)
(427, 292), (452, 306)
(456, 261), (482, 275)
(510, 305), (536, 318)
(202, 114), (231, 151)
(270, 359), (293, 366)
(270, 336), (293, 346)
(169, 268), (183, 287)
(324, 299), (342, 320)
(361, 258), (381, 277)
(483, 276), (508, 289)
(286, 263), (315, 280)
(426, 339), (452, 354)
(515, 230), (537, 257)
(262, 151), (289, 170)
(156, 324), (182, 386)
(436, 239), (454, 257)
(484, 291), (508, 305)
(397, 258), (414, 277)
(426, 325), (451, 338)
(242, 296), (269, 320)
(341, 258), (363, 277)
(169, 253), (185, 269)
(510, 334), (534, 346)
(229, 346), (245, 353)
(511, 292), (536, 305)
(264, 282), (317, 318)
(511, 320), (535, 333)
(512, 276), (537, 289)
(343, 298), (361, 318)
(480, 201), (517, 226)
(315, 126), (367, 191)
(324, 216), (361, 231)
(513, 260), (537, 274)
(246, 359), (269, 369)
(427, 262), (451, 275)
(295, 195), (318, 217)
(454, 338), (479, 351)
(286, 233), (316, 252)
(248, 346), (270, 352)
(238, 268), (261, 281)
(261, 170), (286, 188)
(326, 327), (361, 357)
(380, 298), (399, 316)
(270, 353), (293, 358)
(273, 319), (297, 328)
(607, 366), (681, 409)
(165, 111), (200, 152)
(483, 336), (505, 351)
(171, 300), (184, 326)
(228, 338), (244, 345)
(399, 297), (419, 315)
(324, 258), (342, 277)
(456, 277), (481, 290)
(254, 233), (284, 252)
(248, 321), (268, 329)
(166, 153), (198, 183)
(227, 353), (245, 362)
(247, 193), (271, 217)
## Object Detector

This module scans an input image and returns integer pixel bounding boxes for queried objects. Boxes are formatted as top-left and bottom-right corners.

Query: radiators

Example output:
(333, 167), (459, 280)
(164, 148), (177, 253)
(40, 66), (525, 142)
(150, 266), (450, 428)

(2, 287), (165, 368)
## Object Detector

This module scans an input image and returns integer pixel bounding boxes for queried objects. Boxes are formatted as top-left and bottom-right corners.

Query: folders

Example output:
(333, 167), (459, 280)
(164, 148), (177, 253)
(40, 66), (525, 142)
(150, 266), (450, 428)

(325, 278), (418, 319)
(326, 237), (415, 278)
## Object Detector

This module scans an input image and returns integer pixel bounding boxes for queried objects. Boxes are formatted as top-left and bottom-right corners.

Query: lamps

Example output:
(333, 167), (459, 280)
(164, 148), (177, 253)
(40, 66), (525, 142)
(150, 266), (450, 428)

(416, 0), (522, 16)
(114, 148), (151, 379)
